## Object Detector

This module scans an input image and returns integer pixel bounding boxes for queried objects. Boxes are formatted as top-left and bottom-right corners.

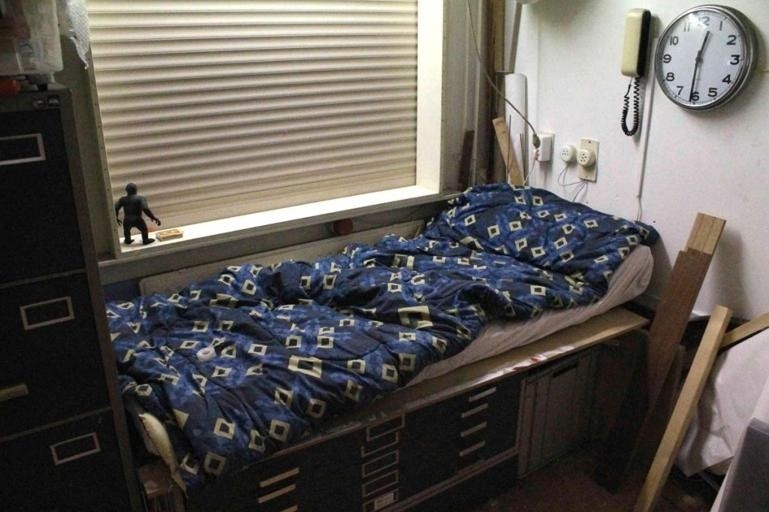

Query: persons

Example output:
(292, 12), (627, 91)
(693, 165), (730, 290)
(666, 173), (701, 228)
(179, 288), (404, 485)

(115, 183), (161, 244)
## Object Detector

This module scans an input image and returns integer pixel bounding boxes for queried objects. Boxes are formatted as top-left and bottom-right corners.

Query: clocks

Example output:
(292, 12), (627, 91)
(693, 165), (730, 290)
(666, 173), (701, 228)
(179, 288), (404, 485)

(653, 5), (758, 112)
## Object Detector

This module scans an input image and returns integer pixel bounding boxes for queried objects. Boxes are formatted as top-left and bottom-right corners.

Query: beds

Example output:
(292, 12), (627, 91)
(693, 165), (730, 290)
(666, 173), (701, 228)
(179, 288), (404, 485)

(104, 182), (653, 511)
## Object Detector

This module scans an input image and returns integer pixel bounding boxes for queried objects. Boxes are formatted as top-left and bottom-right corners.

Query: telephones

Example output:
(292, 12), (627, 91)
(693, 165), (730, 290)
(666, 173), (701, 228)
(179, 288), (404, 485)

(620, 8), (651, 77)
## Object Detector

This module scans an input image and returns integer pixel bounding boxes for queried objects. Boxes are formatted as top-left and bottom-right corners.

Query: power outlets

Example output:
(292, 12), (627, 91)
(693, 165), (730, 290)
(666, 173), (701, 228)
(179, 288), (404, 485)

(532, 137), (551, 163)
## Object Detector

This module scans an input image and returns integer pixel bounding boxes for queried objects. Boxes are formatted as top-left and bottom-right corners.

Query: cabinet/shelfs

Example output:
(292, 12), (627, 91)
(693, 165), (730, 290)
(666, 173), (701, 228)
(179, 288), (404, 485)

(2, 81), (144, 508)
